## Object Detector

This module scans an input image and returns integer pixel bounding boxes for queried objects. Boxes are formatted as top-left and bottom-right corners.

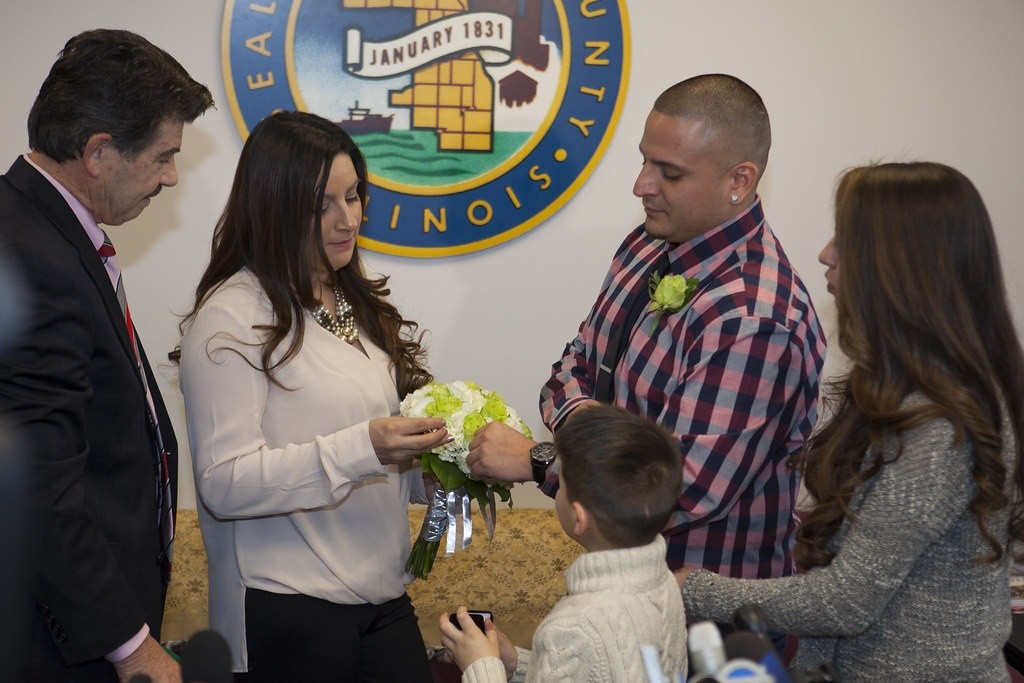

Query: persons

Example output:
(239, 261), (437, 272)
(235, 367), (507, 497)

(674, 162), (1024, 682)
(0, 27), (217, 683)
(177, 108), (486, 681)
(439, 404), (690, 682)
(468, 73), (826, 667)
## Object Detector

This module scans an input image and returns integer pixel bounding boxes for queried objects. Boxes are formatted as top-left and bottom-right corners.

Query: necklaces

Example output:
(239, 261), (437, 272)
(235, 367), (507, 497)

(310, 281), (362, 343)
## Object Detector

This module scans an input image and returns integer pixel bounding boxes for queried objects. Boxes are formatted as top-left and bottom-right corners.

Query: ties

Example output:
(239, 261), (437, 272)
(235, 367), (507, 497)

(97, 230), (173, 587)
(595, 250), (671, 405)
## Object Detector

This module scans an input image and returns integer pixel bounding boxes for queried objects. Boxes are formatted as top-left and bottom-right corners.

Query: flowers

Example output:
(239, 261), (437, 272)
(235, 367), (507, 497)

(644, 273), (696, 316)
(399, 380), (534, 579)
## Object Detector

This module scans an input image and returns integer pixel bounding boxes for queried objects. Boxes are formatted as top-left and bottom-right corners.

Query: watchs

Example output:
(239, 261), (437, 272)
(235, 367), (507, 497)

(530, 441), (559, 486)
